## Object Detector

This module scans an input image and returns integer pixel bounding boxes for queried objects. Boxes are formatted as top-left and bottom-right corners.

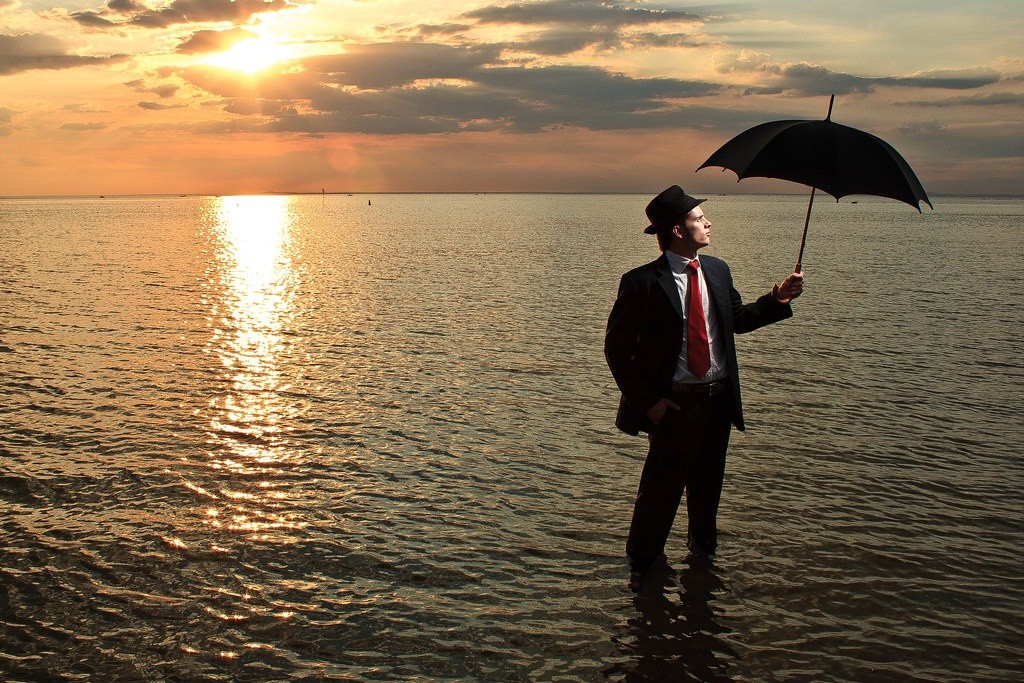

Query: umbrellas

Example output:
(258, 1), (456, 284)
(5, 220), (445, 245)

(696, 95), (933, 305)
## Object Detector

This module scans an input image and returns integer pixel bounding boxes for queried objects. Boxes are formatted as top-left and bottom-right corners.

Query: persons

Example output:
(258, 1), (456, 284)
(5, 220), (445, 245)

(602, 184), (804, 587)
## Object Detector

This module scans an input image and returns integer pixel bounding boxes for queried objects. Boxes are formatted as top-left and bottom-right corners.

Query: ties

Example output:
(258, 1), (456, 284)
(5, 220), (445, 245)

(686, 260), (711, 381)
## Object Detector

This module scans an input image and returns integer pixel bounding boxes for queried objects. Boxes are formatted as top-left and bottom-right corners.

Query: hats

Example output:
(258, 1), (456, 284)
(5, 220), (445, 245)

(644, 184), (708, 235)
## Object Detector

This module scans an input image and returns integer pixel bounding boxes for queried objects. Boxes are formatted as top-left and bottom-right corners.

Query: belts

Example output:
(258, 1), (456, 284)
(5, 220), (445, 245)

(672, 382), (733, 398)
